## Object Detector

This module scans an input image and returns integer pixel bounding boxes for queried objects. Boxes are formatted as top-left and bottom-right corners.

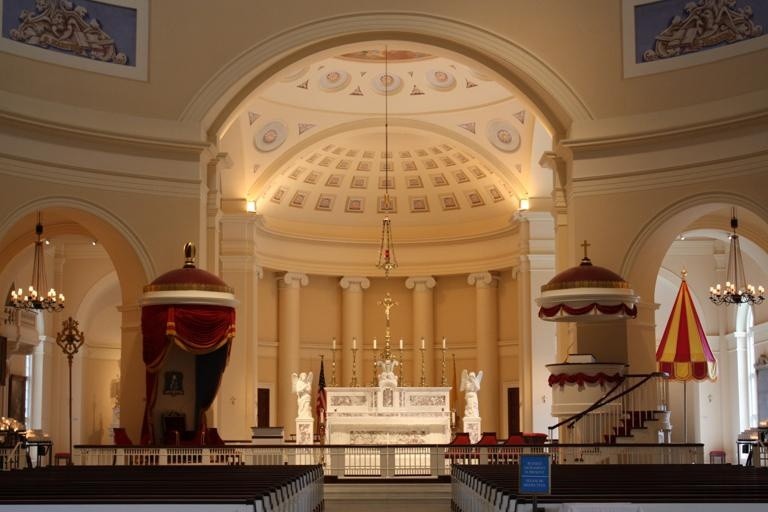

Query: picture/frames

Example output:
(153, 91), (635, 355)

(159, 367), (185, 400)
(8, 374), (27, 448)
(0, 0), (149, 82)
(754, 351), (768, 428)
(622, 0), (768, 80)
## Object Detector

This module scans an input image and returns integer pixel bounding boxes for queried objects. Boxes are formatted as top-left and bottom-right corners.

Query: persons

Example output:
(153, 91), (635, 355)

(457, 368), (485, 419)
(289, 370), (316, 419)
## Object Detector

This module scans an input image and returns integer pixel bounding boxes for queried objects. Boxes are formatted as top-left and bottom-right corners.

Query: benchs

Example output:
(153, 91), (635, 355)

(450, 464), (768, 512)
(0, 465), (325, 512)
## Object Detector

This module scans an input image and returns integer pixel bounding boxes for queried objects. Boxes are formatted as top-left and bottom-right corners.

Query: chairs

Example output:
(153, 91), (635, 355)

(444, 432), (470, 464)
(499, 430), (524, 464)
(471, 432), (498, 465)
(159, 407), (205, 448)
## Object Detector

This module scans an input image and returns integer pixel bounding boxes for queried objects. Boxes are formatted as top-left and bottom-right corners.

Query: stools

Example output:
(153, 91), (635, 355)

(54, 452), (70, 465)
(709, 451), (725, 464)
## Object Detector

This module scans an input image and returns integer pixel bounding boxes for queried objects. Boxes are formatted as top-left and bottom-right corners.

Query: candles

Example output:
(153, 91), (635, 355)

(443, 339), (445, 348)
(332, 340), (335, 349)
(421, 340), (424, 349)
(353, 340), (356, 349)
(373, 339), (376, 349)
(399, 339), (403, 349)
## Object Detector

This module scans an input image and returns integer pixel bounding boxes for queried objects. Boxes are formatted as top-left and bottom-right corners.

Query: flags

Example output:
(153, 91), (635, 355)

(316, 362), (328, 441)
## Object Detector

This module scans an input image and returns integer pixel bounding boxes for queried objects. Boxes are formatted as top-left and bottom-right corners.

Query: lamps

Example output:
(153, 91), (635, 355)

(376, 44), (399, 281)
(11, 210), (65, 313)
(708, 206), (765, 306)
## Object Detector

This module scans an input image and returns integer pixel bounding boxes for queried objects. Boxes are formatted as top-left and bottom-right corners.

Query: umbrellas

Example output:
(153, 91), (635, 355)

(657, 270), (719, 444)
